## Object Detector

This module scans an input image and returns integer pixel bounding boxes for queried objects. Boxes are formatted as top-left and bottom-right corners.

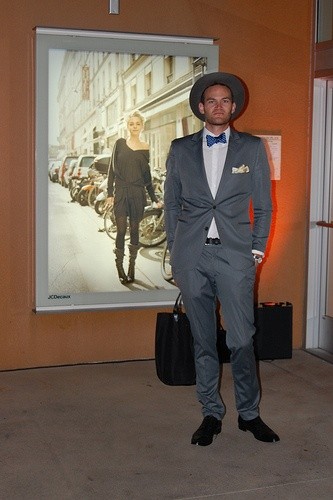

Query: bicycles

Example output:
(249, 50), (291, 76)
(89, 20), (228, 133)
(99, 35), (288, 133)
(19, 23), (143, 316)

(69, 168), (175, 281)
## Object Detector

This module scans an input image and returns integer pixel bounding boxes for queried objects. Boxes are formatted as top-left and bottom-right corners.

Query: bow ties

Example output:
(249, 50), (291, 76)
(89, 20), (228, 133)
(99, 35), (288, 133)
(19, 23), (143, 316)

(206, 132), (227, 147)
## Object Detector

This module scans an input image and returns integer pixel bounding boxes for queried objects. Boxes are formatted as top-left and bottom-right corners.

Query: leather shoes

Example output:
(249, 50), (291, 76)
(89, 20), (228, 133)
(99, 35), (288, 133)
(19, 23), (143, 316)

(238, 414), (279, 442)
(192, 415), (222, 446)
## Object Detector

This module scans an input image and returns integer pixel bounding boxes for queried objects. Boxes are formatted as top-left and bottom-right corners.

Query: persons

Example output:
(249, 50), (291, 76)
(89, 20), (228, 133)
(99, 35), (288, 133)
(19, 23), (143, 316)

(103, 111), (162, 285)
(164, 72), (280, 447)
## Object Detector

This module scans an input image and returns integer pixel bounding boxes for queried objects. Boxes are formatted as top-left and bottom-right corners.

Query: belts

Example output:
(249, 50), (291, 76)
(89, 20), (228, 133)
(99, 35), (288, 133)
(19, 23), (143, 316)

(204, 237), (221, 246)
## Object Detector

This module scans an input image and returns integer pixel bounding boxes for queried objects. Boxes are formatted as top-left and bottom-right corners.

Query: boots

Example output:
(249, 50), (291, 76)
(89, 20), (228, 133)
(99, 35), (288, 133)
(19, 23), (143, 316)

(127, 243), (140, 282)
(114, 248), (128, 283)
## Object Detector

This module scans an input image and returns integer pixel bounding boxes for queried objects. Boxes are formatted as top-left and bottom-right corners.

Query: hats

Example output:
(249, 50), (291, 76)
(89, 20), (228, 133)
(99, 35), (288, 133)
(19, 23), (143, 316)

(189, 72), (245, 122)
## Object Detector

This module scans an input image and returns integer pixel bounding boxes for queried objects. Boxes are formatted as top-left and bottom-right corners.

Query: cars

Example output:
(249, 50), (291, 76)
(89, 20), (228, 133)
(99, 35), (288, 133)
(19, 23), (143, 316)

(48, 154), (111, 188)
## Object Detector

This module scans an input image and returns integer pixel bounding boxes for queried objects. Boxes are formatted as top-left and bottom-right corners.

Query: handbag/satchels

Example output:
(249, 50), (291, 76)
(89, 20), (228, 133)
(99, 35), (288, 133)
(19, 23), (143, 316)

(155, 312), (196, 387)
